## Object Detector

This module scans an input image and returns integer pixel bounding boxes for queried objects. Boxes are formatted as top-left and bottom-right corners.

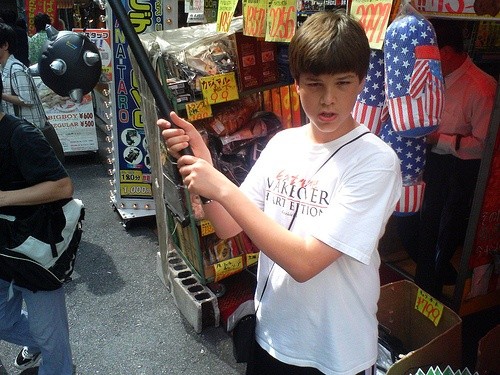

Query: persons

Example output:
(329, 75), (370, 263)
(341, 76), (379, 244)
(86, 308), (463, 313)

(0, 8), (54, 128)
(400, 17), (497, 298)
(155, 9), (405, 375)
(0, 76), (74, 375)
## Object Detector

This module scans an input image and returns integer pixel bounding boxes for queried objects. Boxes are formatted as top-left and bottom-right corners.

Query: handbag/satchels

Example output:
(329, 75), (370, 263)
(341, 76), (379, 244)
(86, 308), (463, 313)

(39, 121), (65, 162)
(0, 192), (86, 294)
(226, 299), (257, 366)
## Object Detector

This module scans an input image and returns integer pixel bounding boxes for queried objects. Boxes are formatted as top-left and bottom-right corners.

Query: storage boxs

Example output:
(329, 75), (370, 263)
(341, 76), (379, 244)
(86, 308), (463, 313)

(375, 280), (462, 375)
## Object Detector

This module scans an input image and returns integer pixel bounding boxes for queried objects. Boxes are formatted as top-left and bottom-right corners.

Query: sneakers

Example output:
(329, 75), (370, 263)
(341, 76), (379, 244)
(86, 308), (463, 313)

(14, 347), (42, 370)
